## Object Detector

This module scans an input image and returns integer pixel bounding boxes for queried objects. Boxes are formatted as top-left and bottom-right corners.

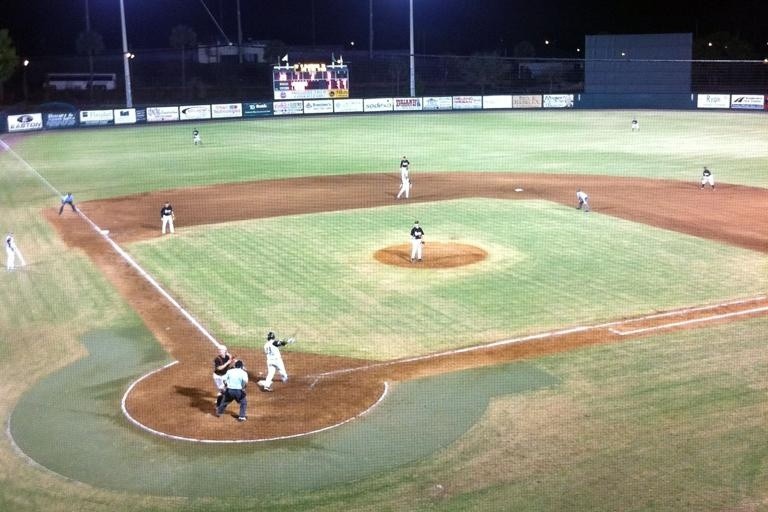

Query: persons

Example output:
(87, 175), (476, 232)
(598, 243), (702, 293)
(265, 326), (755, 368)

(632, 117), (638, 131)
(160, 201), (175, 235)
(410, 220), (424, 261)
(400, 155), (410, 178)
(701, 166), (715, 190)
(5, 232), (16, 271)
(59, 192), (75, 214)
(215, 360), (247, 422)
(212, 344), (247, 410)
(397, 175), (412, 199)
(193, 127), (202, 145)
(263, 331), (295, 391)
(576, 189), (589, 211)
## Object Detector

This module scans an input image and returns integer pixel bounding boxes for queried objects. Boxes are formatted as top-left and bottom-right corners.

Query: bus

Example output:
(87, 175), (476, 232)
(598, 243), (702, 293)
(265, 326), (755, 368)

(41, 72), (121, 93)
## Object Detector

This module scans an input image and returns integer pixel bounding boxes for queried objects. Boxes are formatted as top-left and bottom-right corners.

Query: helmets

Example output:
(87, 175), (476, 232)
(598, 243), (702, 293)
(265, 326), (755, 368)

(267, 331), (275, 339)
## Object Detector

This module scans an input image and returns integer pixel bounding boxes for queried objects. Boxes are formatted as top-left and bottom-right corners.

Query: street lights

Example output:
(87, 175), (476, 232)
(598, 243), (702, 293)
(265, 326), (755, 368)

(22, 59), (31, 106)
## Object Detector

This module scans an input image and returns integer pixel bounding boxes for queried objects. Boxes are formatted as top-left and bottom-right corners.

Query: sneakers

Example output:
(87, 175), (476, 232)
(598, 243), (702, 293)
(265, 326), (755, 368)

(238, 415), (249, 421)
(263, 387), (274, 392)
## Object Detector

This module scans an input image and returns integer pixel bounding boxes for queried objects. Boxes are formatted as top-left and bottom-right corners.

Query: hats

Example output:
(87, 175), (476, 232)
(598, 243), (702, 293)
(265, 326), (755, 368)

(216, 344), (228, 351)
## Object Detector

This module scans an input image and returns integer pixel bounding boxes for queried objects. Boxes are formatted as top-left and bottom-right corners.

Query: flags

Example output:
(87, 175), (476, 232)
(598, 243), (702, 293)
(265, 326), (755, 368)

(282, 53), (289, 62)
(332, 53), (336, 62)
